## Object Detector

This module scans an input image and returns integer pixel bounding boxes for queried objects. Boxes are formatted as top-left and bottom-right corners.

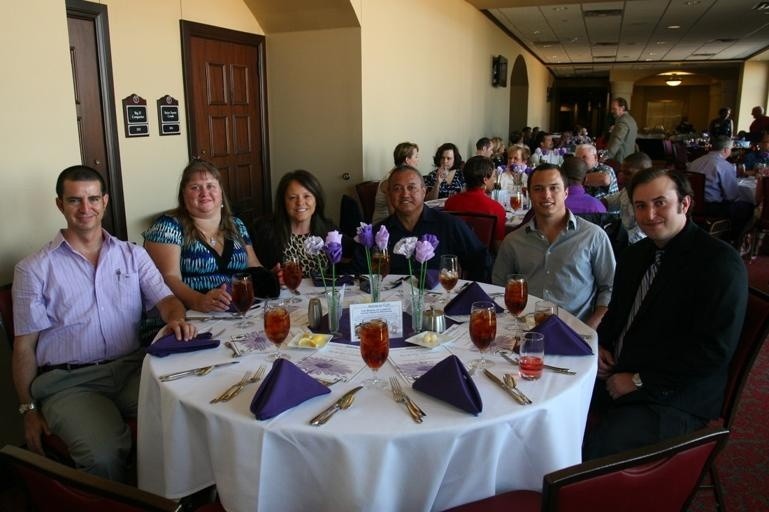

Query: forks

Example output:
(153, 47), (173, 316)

(208, 367), (265, 404)
(318, 378), (342, 386)
(224, 342), (242, 358)
(499, 352), (576, 376)
(388, 376), (426, 416)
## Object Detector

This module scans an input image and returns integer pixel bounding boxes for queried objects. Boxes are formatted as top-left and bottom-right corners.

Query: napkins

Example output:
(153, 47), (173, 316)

(311, 271), (355, 288)
(513, 314), (594, 357)
(145, 331), (220, 359)
(443, 281), (506, 315)
(412, 353), (483, 418)
(250, 358), (331, 422)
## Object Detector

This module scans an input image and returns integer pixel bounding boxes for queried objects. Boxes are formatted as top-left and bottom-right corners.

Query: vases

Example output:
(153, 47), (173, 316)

(410, 294), (423, 333)
(368, 273), (382, 302)
(324, 290), (340, 332)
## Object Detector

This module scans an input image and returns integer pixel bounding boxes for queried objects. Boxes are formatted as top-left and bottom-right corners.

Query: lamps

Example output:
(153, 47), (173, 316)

(665, 74), (682, 87)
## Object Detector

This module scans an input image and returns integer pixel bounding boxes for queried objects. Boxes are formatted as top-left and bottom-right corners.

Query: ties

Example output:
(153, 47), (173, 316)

(612, 250), (666, 367)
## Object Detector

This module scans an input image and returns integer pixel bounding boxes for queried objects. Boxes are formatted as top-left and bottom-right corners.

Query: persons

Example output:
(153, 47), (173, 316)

(490, 163), (617, 328)
(357, 167), (491, 284)
(141, 156), (285, 344)
(373, 98), (769, 258)
(591, 165), (749, 458)
(255, 167), (354, 280)
(11, 166), (197, 483)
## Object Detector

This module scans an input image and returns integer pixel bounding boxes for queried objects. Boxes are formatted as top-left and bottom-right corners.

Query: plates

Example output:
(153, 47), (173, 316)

(287, 331), (335, 350)
(404, 330), (453, 349)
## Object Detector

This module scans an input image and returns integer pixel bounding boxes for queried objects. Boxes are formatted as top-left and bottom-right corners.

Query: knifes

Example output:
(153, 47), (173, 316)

(482, 367), (526, 406)
(310, 385), (364, 426)
(184, 315), (242, 321)
(159, 361), (239, 379)
(391, 275), (411, 289)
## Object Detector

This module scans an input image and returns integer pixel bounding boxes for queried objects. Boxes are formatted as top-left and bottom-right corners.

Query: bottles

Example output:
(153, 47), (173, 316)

(423, 306), (446, 332)
(308, 299), (322, 329)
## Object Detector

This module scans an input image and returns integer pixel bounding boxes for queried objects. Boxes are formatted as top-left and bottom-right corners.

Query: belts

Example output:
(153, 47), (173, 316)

(36, 349), (145, 373)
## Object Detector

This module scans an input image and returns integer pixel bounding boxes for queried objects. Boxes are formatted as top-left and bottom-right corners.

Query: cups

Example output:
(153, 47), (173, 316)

(738, 163), (746, 178)
(519, 332), (545, 381)
(534, 301), (559, 326)
(441, 166), (449, 178)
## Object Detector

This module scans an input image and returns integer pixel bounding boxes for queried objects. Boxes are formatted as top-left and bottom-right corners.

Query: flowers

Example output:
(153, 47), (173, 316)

(303, 230), (345, 293)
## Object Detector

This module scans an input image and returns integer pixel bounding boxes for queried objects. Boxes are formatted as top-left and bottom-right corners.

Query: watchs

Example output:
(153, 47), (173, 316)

(17, 401), (38, 416)
(630, 371), (643, 387)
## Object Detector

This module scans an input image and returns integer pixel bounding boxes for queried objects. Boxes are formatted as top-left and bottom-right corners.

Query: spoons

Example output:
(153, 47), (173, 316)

(318, 394), (354, 424)
(161, 366), (213, 382)
(503, 373), (531, 403)
(393, 388), (423, 423)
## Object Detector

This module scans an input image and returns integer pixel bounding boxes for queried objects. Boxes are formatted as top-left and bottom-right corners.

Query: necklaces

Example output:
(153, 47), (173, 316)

(199, 223), (219, 247)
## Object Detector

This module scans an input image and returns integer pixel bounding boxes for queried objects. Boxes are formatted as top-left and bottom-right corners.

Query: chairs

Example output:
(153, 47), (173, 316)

(579, 286), (768, 508)
(338, 132), (769, 288)
(3, 442), (226, 509)
(3, 283), (72, 458)
(448, 428), (732, 508)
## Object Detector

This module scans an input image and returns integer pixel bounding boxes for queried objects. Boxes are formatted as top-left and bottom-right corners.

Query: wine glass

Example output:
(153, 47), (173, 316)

(372, 246), (390, 291)
(508, 191), (522, 216)
(360, 317), (390, 389)
(264, 297), (292, 362)
(506, 273), (529, 330)
(440, 254), (460, 304)
(233, 273), (256, 329)
(470, 301), (496, 369)
(282, 255), (304, 304)
